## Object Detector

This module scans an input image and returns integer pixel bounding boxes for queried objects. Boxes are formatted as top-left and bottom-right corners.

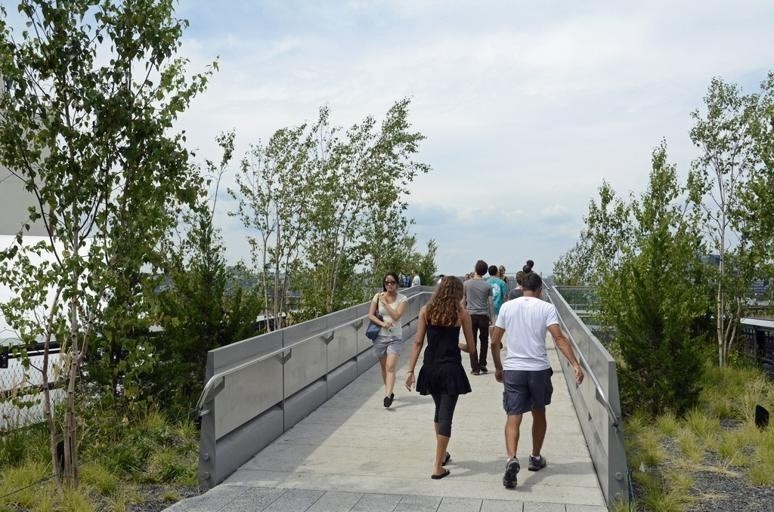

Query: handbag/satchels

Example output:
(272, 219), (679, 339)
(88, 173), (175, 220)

(366, 310), (383, 339)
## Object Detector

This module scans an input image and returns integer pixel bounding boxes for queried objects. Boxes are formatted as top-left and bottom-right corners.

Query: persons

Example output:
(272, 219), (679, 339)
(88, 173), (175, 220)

(460, 259), (495, 376)
(368, 270), (408, 408)
(402, 274), (480, 479)
(488, 271), (585, 487)
(397, 259), (544, 350)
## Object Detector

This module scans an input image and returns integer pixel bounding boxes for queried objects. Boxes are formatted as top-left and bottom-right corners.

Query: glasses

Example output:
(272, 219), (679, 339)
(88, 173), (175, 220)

(385, 281), (395, 285)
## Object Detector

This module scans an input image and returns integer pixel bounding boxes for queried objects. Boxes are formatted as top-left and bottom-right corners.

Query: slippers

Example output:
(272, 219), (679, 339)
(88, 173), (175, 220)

(432, 469), (449, 478)
(442, 454), (450, 466)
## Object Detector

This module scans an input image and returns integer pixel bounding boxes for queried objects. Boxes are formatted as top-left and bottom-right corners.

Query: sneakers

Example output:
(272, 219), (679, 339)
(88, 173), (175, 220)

(503, 457), (520, 487)
(384, 393), (394, 407)
(472, 364), (487, 374)
(529, 454), (546, 471)
